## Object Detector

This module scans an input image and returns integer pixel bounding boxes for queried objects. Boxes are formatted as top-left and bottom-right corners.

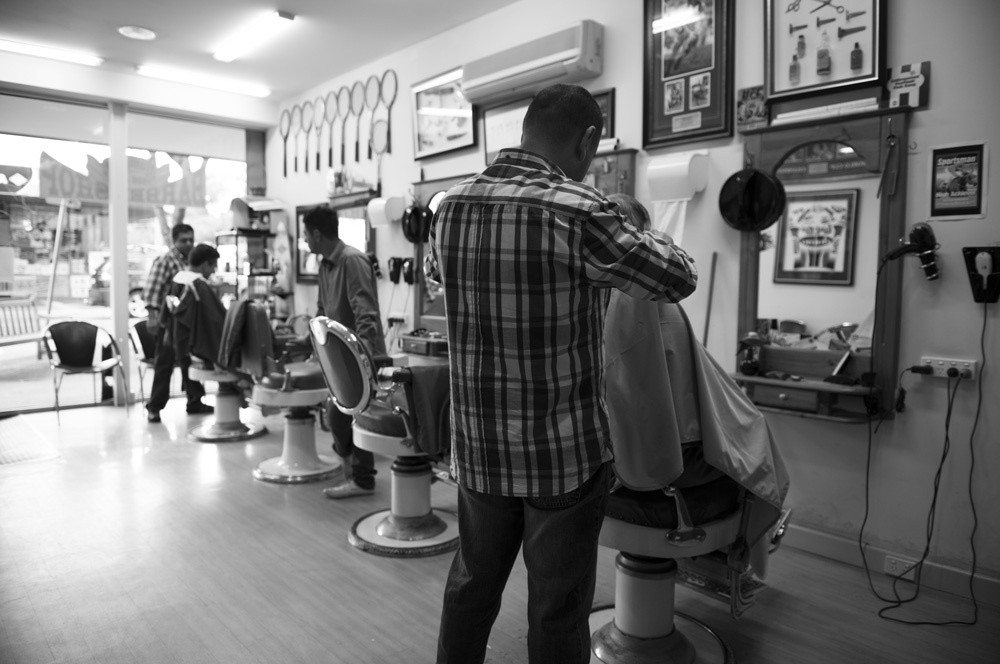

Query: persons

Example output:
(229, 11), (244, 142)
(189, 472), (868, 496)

(287, 205), (388, 496)
(600, 194), (789, 585)
(427, 84), (698, 663)
(143, 223), (214, 423)
(169, 244), (227, 360)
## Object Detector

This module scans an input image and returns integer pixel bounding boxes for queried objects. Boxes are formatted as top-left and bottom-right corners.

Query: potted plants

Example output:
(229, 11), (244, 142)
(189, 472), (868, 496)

(459, 20), (604, 108)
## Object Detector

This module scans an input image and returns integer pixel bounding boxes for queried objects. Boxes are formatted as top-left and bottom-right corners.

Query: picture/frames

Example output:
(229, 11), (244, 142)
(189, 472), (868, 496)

(591, 87), (615, 138)
(642, 0), (735, 150)
(924, 138), (986, 221)
(411, 66), (477, 162)
(763, 0), (887, 107)
(772, 189), (859, 286)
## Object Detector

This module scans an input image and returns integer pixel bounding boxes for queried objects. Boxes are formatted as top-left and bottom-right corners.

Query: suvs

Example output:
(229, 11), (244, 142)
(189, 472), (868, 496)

(87, 249), (166, 320)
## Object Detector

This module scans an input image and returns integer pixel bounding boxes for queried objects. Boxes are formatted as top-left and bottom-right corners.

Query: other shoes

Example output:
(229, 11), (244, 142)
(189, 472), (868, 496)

(147, 408), (161, 422)
(186, 403), (214, 413)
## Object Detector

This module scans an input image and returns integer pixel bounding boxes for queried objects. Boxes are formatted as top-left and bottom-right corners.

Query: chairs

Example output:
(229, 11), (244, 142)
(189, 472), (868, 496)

(309, 316), (460, 558)
(43, 320), (129, 425)
(589, 375), (749, 664)
(160, 271), (265, 443)
(217, 298), (342, 484)
(127, 320), (153, 403)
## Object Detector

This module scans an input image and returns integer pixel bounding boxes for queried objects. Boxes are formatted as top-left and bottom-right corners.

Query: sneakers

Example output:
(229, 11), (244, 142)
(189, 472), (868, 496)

(324, 482), (374, 498)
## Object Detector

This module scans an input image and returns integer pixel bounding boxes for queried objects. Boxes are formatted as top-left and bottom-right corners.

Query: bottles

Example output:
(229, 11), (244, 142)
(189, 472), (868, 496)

(850, 42), (862, 69)
(797, 35), (805, 56)
(789, 55), (799, 82)
(816, 30), (831, 76)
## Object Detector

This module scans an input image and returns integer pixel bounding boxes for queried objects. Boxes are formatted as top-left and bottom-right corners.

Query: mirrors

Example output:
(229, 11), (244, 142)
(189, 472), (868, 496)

(740, 106), (909, 425)
(295, 205), (368, 284)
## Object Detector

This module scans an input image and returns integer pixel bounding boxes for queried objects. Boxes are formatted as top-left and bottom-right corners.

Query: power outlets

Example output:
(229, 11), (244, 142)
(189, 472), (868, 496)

(920, 356), (975, 381)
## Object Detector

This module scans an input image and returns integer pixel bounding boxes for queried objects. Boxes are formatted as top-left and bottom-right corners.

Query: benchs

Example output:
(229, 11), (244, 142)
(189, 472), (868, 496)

(0, 301), (52, 359)
